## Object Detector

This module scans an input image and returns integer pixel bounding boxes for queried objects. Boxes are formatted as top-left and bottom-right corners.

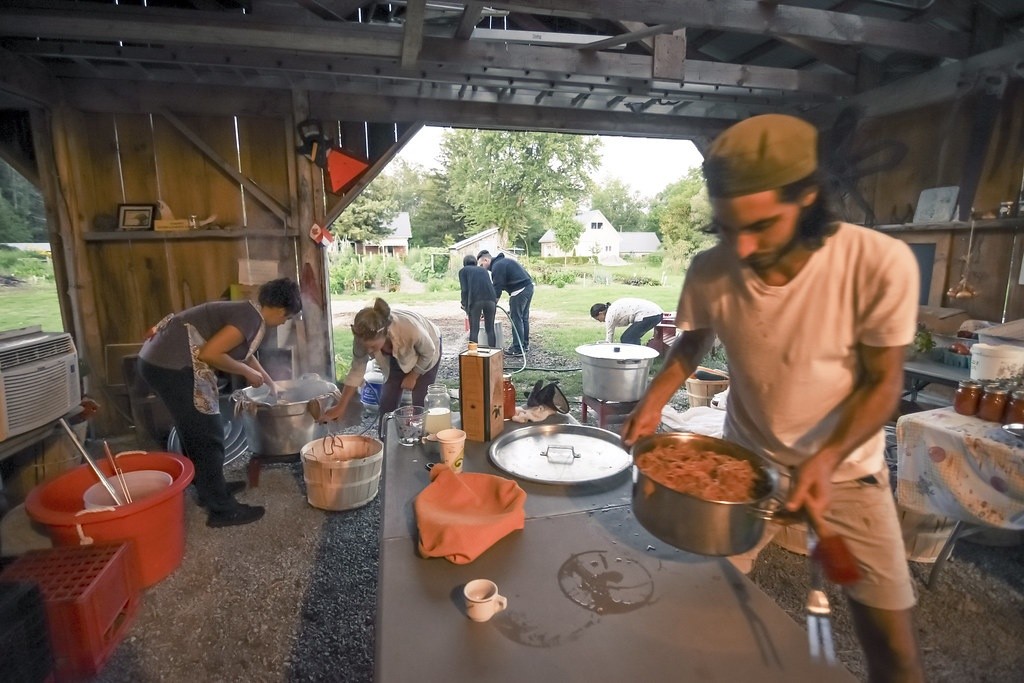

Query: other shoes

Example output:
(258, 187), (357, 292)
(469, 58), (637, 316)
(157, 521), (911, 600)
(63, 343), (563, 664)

(207, 503), (265, 528)
(198, 481), (247, 505)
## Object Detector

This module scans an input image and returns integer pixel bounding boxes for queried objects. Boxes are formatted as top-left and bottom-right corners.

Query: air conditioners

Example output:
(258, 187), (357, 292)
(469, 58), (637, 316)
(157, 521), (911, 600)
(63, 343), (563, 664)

(0, 331), (81, 442)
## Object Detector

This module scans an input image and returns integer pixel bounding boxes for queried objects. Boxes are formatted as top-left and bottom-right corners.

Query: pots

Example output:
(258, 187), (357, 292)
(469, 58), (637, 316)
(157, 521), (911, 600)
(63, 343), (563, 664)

(574, 339), (660, 403)
(230, 371), (339, 456)
(622, 434), (787, 558)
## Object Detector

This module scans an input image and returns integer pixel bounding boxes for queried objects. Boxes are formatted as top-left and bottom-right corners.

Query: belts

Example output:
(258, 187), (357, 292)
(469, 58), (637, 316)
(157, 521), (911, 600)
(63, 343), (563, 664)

(860, 475), (878, 484)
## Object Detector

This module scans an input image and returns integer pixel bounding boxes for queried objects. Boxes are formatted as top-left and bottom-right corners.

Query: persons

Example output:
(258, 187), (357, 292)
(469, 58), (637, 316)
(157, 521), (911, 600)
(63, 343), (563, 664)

(459, 255), (497, 347)
(477, 250), (533, 356)
(623, 112), (921, 682)
(316, 298), (443, 442)
(590, 297), (664, 345)
(140, 278), (302, 525)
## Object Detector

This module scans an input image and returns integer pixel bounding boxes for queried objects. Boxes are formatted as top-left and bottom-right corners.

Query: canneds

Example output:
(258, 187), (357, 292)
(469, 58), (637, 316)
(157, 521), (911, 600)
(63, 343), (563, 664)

(999, 201), (1014, 218)
(954, 378), (1024, 426)
(503, 375), (515, 420)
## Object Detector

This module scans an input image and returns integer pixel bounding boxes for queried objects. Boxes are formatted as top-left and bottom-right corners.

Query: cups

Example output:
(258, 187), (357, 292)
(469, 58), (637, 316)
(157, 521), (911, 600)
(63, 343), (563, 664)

(380, 406), (429, 447)
(436, 428), (468, 475)
(464, 579), (508, 623)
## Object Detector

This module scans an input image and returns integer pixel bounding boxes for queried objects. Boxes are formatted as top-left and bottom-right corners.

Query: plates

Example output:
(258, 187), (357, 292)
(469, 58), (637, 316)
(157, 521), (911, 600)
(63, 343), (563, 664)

(1001, 422), (1024, 442)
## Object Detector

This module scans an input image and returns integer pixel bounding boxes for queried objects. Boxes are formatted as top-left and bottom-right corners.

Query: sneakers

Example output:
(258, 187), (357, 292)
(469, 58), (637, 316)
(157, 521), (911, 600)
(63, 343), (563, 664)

(502, 344), (529, 356)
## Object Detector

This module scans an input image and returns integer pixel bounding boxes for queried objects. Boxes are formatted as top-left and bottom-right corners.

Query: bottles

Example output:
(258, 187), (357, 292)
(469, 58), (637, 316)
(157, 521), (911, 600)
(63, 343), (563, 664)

(189, 215), (199, 230)
(424, 384), (452, 438)
(502, 373), (516, 421)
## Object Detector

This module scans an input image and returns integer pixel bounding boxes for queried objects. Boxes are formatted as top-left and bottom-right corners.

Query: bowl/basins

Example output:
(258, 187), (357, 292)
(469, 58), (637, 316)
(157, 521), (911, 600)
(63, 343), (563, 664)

(299, 434), (384, 512)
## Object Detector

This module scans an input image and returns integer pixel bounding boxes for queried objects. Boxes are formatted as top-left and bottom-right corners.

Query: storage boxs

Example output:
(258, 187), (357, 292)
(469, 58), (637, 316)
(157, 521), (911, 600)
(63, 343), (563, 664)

(459, 346), (504, 443)
(0, 541), (139, 683)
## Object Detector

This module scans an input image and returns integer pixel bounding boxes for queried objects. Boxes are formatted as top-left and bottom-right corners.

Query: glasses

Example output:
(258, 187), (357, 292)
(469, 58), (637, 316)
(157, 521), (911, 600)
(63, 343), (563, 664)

(350, 324), (385, 338)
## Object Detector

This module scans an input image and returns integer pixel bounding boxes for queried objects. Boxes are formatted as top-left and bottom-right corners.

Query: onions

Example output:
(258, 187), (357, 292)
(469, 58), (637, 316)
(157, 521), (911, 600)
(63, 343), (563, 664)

(947, 284), (976, 300)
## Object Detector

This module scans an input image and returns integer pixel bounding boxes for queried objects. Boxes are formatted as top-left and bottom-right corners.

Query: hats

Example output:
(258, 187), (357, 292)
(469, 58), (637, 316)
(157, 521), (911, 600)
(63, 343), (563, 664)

(703, 112), (817, 198)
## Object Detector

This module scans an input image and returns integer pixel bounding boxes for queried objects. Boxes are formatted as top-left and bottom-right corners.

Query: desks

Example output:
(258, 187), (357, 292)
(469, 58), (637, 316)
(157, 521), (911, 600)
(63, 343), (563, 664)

(900, 359), (970, 410)
(377, 406), (860, 683)
(895, 406), (1024, 587)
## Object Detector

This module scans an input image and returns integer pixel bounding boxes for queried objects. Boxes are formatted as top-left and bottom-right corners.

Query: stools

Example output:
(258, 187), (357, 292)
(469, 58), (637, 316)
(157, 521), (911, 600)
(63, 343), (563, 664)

(580, 394), (639, 428)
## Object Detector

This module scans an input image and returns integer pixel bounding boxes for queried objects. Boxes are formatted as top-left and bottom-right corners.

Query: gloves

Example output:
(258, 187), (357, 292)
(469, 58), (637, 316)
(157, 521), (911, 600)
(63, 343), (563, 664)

(524, 380), (570, 414)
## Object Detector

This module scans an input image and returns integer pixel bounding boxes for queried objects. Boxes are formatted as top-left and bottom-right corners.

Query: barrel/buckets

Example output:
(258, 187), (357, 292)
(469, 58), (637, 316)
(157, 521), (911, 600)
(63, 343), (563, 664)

(24, 449), (195, 589)
(683, 376), (731, 408)
